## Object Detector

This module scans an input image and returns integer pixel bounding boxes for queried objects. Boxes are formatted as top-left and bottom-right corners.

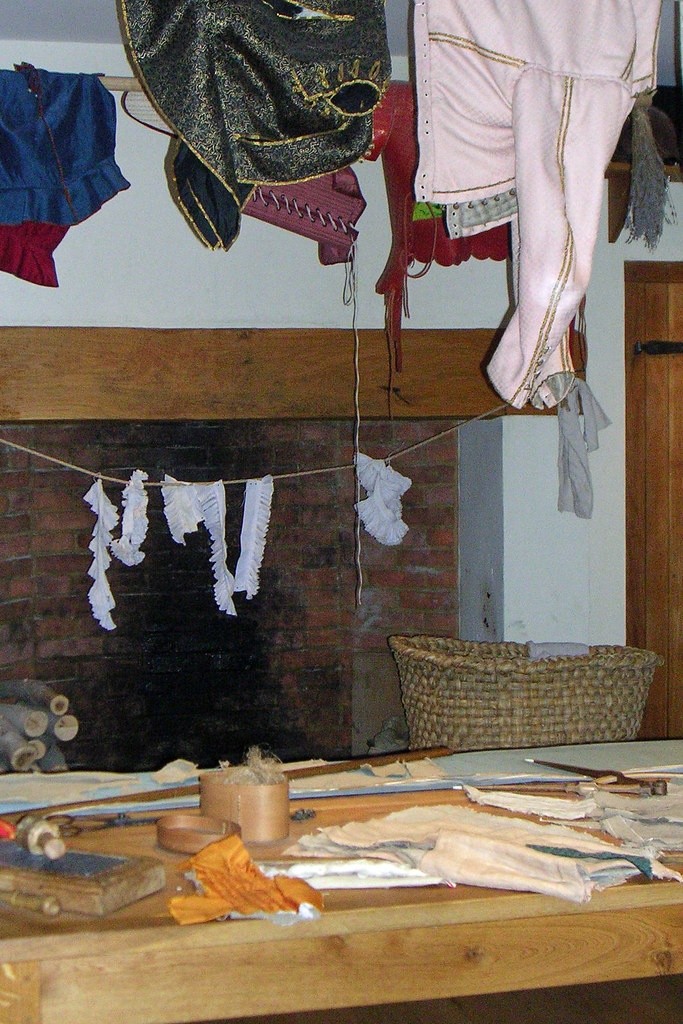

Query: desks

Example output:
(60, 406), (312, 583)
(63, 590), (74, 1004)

(0, 736), (683, 1024)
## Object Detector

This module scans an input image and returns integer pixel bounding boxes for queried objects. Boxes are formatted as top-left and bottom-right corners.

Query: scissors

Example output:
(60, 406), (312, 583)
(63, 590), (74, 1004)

(44, 814), (162, 840)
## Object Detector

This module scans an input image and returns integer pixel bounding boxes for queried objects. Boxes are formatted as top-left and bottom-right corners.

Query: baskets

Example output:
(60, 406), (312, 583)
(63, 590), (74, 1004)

(387, 634), (666, 752)
